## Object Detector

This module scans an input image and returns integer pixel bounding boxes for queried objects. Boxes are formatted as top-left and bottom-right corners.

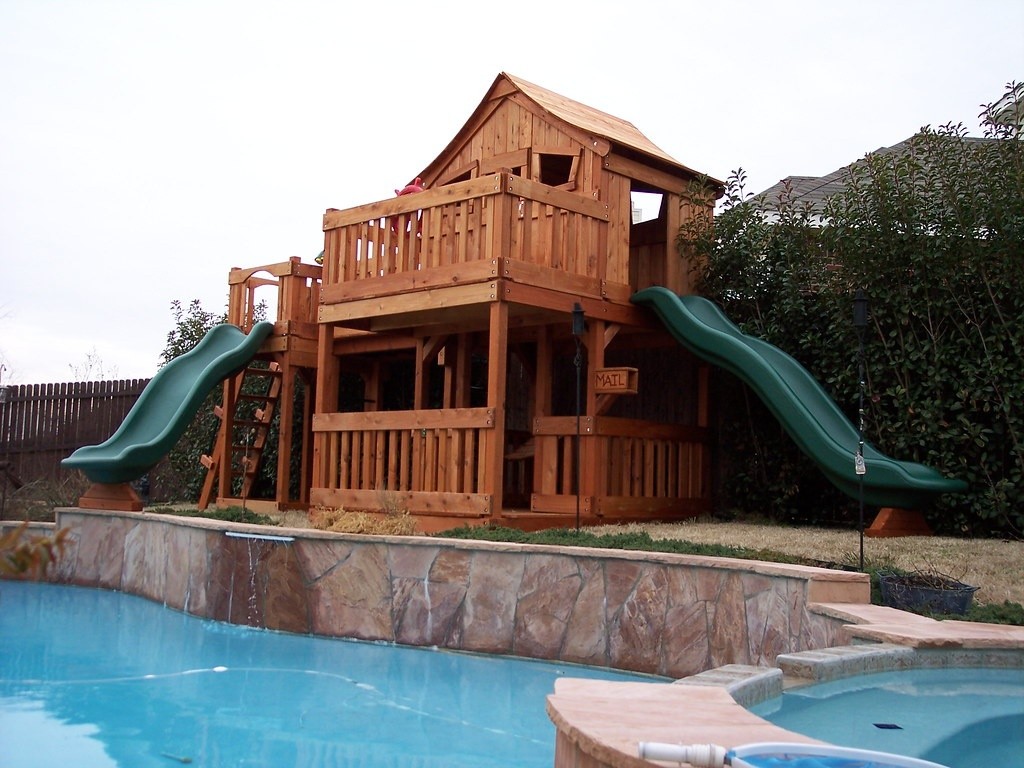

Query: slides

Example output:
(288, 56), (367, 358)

(59, 320), (273, 484)
(633, 285), (969, 507)
